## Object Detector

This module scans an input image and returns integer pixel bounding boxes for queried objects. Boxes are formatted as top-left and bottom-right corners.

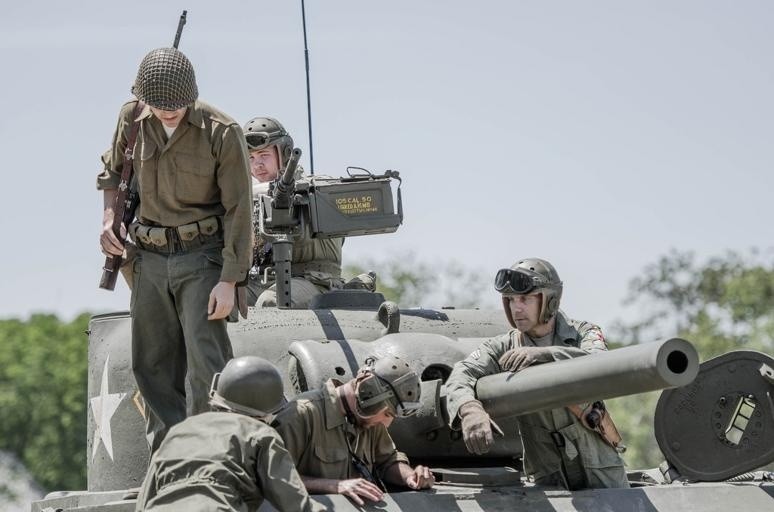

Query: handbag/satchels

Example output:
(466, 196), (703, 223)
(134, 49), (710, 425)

(126, 216), (224, 256)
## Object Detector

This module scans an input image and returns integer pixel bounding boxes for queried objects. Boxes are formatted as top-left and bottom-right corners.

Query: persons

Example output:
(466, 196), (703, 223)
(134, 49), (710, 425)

(271, 355), (436, 505)
(99, 48), (253, 462)
(443, 258), (633, 488)
(134, 355), (328, 512)
(243, 116), (345, 308)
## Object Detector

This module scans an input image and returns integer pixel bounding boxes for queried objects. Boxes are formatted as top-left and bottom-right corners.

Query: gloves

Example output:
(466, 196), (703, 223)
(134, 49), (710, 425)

(498, 346), (554, 374)
(457, 399), (505, 456)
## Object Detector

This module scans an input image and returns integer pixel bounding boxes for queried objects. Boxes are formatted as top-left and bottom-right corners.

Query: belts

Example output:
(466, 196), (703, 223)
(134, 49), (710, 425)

(255, 259), (343, 279)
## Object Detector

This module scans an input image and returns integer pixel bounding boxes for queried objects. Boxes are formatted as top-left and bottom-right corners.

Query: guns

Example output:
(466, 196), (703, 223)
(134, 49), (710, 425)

(98, 11), (187, 290)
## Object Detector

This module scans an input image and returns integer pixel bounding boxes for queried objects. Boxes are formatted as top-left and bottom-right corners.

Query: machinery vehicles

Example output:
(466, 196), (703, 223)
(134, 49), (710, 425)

(22, 142), (772, 512)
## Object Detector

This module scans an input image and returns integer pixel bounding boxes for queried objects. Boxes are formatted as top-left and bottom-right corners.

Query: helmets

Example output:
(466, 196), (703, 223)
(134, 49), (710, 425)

(129, 46), (199, 112)
(502, 257), (563, 329)
(354, 353), (423, 421)
(242, 115), (294, 172)
(208, 355), (288, 420)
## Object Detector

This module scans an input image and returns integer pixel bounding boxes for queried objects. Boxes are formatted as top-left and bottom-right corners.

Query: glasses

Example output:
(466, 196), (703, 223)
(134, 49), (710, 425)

(243, 131), (282, 150)
(494, 268), (545, 295)
(396, 400), (425, 420)
